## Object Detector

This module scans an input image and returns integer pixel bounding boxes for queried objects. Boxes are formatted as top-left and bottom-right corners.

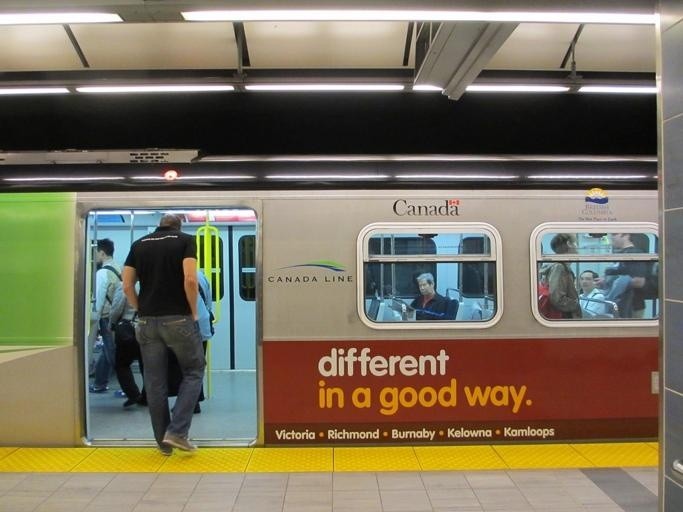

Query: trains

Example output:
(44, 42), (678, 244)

(0, 161), (661, 447)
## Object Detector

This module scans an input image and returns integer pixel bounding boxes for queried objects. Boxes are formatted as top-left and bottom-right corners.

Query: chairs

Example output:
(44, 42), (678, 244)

(367, 288), (494, 321)
(579, 297), (619, 318)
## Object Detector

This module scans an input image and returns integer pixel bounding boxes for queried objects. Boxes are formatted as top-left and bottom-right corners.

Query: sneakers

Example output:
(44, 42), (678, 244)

(161, 447), (173, 456)
(89, 384), (110, 393)
(171, 401), (201, 414)
(113, 388), (127, 399)
(162, 429), (199, 451)
(123, 394), (148, 408)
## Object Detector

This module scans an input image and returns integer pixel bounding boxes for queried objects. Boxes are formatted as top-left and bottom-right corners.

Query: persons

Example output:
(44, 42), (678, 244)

(88, 237), (127, 400)
(538, 231), (658, 320)
(170, 269), (216, 417)
(106, 279), (150, 409)
(406, 271), (449, 320)
(118, 212), (207, 457)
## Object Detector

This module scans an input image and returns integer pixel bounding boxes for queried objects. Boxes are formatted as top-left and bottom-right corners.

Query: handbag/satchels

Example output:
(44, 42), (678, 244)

(209, 311), (216, 335)
(114, 319), (134, 348)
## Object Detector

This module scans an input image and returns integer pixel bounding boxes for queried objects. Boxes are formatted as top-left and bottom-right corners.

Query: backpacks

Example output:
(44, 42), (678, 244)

(537, 262), (568, 319)
